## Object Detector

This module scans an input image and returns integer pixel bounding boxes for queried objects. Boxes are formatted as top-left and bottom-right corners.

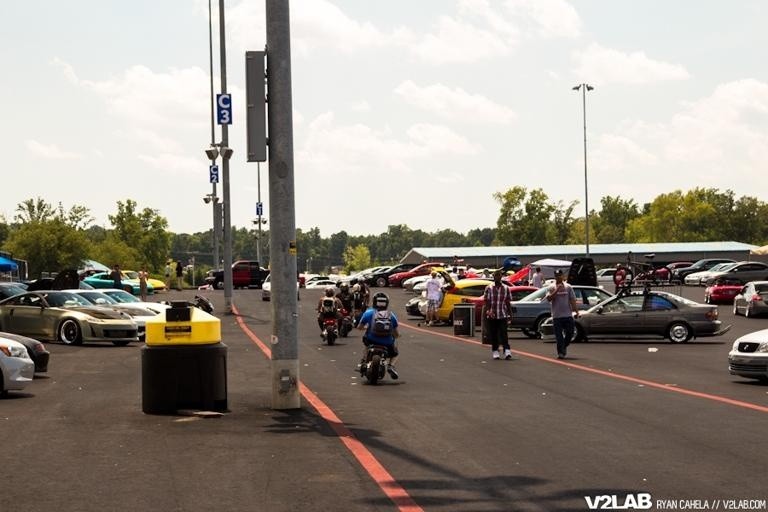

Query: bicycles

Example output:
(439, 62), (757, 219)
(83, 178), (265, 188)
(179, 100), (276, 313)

(613, 251), (672, 285)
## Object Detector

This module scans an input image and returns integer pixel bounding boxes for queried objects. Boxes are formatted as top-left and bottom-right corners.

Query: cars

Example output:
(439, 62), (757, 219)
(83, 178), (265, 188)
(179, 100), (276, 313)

(733, 281), (768, 317)
(405, 279), (540, 325)
(596, 268), (632, 282)
(352, 263), (448, 287)
(77, 259), (111, 281)
(0, 291), (140, 345)
(402, 268), (466, 295)
(83, 271), (165, 295)
(510, 286), (627, 338)
(729, 330), (768, 377)
(647, 258), (768, 285)
(0, 338), (36, 398)
(262, 273), (300, 302)
(1, 332), (50, 373)
(539, 291), (733, 344)
(26, 278), (95, 290)
(61, 289), (172, 337)
(305, 276), (336, 289)
(475, 268), (514, 277)
(0, 282), (29, 301)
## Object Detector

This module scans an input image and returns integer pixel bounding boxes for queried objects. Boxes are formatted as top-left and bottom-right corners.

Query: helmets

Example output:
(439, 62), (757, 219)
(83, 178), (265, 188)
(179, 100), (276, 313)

(324, 287), (335, 297)
(372, 292), (389, 310)
(358, 277), (365, 284)
(340, 283), (349, 293)
(352, 283), (360, 292)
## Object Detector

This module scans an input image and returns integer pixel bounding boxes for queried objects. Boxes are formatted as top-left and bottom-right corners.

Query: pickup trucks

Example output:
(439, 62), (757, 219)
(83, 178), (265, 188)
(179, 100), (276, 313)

(205, 260), (271, 289)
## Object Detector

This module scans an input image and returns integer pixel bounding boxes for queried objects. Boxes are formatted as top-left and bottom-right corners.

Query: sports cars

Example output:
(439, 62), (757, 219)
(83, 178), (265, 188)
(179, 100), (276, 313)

(704, 277), (744, 304)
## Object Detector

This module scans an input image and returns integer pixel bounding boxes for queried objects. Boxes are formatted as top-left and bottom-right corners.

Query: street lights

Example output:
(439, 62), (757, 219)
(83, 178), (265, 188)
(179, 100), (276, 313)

(572, 83), (595, 256)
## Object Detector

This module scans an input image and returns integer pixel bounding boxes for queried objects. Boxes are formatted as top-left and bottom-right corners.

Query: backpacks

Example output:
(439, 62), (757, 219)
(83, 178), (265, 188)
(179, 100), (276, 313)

(322, 298), (335, 313)
(371, 310), (393, 336)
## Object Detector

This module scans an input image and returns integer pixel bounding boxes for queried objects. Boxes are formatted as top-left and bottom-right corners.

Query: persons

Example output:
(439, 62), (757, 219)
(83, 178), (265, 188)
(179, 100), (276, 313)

(548, 269), (579, 359)
(110, 265), (121, 289)
(613, 265), (626, 294)
(458, 269), (467, 280)
(484, 273), (514, 360)
(165, 262), (173, 290)
(450, 267), (458, 280)
(357, 292), (400, 379)
(531, 266), (545, 288)
(315, 289), (344, 333)
(425, 271), (447, 326)
(336, 284), (356, 321)
(176, 260), (183, 290)
(482, 270), (494, 278)
(332, 281), (343, 296)
(139, 267), (148, 302)
(353, 278), (372, 306)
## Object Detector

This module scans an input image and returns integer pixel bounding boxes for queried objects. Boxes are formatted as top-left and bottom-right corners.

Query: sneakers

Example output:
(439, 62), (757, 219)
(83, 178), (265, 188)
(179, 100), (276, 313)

(557, 354), (562, 358)
(387, 366), (399, 380)
(504, 349), (511, 359)
(491, 350), (501, 360)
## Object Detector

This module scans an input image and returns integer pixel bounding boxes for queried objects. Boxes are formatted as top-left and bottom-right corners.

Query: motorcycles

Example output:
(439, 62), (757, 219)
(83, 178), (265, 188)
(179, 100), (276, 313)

(354, 326), (400, 384)
(318, 291), (368, 346)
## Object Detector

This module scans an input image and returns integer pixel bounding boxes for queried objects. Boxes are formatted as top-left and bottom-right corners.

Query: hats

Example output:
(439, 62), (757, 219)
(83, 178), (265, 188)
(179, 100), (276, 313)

(554, 269), (563, 277)
